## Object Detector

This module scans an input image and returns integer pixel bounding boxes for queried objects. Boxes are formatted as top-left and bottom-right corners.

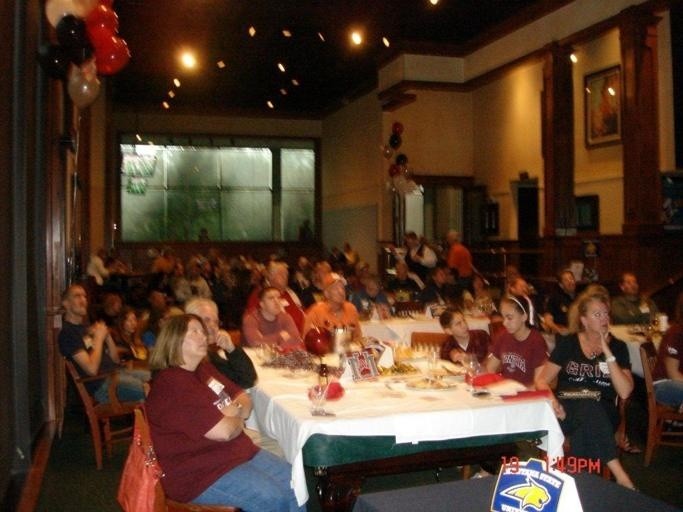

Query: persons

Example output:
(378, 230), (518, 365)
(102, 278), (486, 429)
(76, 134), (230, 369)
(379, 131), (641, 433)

(58, 231), (683, 495)
(139, 314), (305, 511)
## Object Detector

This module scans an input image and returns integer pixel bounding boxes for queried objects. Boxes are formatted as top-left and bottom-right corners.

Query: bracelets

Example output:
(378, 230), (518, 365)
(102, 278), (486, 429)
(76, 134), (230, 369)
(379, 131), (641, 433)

(607, 357), (617, 361)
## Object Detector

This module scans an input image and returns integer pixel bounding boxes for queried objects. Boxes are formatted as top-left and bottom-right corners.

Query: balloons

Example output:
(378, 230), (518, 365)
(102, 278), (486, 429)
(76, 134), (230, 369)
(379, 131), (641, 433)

(383, 121), (416, 193)
(34, 0), (131, 112)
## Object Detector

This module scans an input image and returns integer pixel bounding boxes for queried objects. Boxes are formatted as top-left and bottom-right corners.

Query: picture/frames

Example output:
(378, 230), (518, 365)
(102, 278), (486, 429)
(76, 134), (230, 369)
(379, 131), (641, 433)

(575, 195), (599, 232)
(583, 64), (624, 149)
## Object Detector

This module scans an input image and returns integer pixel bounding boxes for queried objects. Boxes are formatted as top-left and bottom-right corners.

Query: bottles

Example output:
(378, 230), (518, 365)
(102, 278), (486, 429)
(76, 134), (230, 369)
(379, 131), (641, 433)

(657, 315), (669, 333)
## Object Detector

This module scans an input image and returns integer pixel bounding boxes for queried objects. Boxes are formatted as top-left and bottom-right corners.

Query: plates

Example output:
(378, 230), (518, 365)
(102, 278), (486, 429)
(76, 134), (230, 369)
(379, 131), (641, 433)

(344, 350), (451, 395)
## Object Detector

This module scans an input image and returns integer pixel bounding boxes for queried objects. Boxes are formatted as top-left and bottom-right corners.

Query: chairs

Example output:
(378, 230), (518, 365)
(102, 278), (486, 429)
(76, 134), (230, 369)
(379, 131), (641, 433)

(65, 298), (683, 511)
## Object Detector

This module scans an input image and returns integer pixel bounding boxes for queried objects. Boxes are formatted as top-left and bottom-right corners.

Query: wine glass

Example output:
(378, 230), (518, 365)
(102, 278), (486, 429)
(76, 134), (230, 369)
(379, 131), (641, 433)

(460, 353), (480, 396)
(256, 341), (328, 418)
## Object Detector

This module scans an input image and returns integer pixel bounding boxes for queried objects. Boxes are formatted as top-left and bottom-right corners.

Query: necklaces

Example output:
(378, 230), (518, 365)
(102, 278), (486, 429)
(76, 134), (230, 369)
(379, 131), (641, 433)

(590, 347), (600, 360)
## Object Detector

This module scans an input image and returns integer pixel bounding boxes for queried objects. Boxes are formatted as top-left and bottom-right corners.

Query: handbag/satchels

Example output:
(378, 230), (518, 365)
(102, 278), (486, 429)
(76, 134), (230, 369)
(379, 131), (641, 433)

(117, 443), (166, 511)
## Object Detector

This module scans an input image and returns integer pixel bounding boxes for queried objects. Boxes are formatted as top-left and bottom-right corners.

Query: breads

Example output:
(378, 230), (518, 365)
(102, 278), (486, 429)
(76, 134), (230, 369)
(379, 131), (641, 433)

(439, 359), (461, 375)
(410, 379), (447, 389)
